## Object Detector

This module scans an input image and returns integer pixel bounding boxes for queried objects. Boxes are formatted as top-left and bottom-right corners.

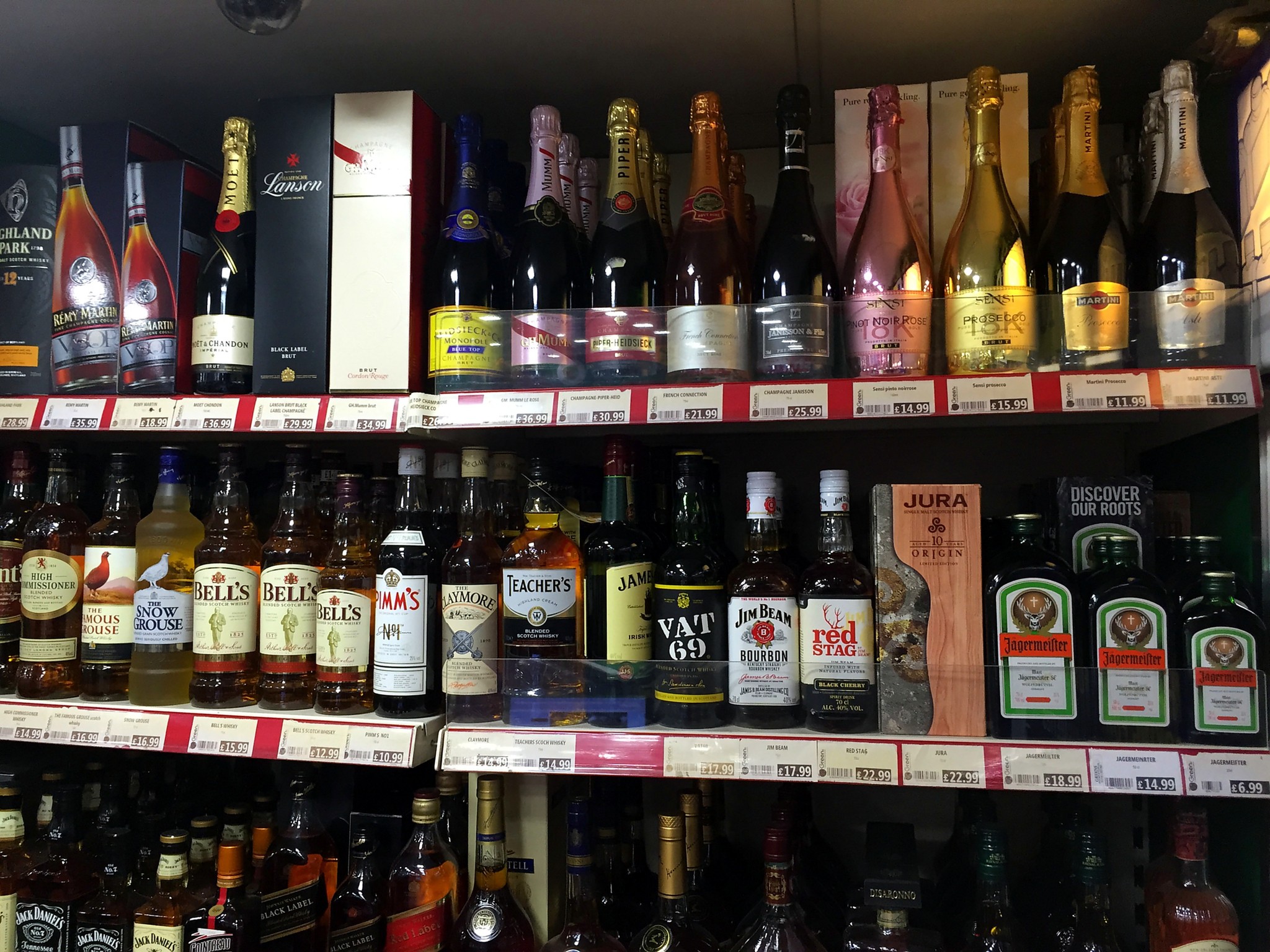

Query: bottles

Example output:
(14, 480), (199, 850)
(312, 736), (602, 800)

(327, 822), (385, 952)
(538, 774), (1121, 952)
(838, 83), (935, 382)
(0, 438), (875, 736)
(121, 161), (177, 394)
(0, 741), (335, 952)
(434, 770), (468, 915)
(192, 115), (257, 397)
(51, 124), (121, 396)
(381, 788), (459, 952)
(424, 90), (761, 387)
(984, 512), (1270, 748)
(448, 773), (535, 952)
(754, 82), (836, 382)
(1035, 59), (1240, 375)
(1142, 795), (1240, 952)
(940, 61), (1041, 375)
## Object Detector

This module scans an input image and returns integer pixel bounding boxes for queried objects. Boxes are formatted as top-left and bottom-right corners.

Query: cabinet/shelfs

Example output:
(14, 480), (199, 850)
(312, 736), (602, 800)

(0, 366), (1270, 797)
